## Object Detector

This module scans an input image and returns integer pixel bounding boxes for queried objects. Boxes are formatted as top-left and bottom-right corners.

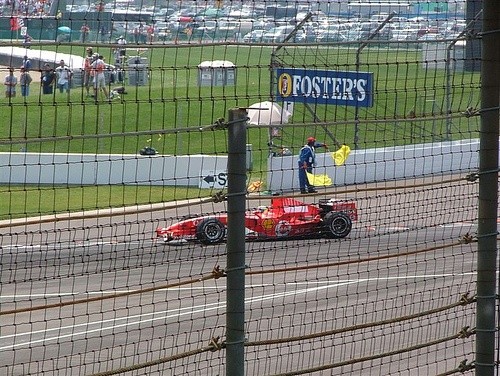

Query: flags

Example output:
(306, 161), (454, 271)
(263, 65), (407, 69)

(331, 144), (350, 165)
(306, 171), (332, 186)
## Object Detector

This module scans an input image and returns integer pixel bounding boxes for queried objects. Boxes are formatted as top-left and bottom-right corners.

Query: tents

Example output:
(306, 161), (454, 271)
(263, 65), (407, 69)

(424, 41), (467, 73)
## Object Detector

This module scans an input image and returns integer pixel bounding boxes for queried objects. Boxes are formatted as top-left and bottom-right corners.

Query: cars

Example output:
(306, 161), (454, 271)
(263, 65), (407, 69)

(62, 1), (467, 50)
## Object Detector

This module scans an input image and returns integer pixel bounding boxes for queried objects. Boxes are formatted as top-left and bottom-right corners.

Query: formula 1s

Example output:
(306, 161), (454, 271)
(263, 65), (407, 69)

(154, 193), (359, 247)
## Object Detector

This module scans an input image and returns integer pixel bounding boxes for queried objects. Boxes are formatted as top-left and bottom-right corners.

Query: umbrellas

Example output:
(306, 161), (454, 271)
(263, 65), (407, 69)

(244, 101), (292, 125)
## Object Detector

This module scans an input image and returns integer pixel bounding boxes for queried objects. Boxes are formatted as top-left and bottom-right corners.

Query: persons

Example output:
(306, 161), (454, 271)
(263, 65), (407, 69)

(90, 56), (110, 103)
(93, 52), (99, 60)
(185, 17), (194, 44)
(22, 56), (31, 71)
(0, 0), (54, 18)
(97, 2), (105, 12)
(83, 46), (94, 97)
(41, 63), (55, 94)
(19, 66), (32, 96)
(4, 67), (17, 98)
(57, 9), (62, 20)
(298, 137), (329, 193)
(117, 34), (127, 56)
(54, 60), (73, 93)
(85, 24), (90, 42)
(80, 23), (86, 43)
(24, 34), (32, 48)
(133, 23), (154, 45)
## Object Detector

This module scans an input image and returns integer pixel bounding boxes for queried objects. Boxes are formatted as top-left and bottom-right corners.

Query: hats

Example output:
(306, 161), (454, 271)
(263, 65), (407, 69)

(308, 137), (315, 142)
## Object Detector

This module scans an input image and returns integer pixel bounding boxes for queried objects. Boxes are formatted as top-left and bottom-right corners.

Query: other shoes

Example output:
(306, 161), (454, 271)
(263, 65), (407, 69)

(309, 190), (318, 193)
(301, 191), (308, 194)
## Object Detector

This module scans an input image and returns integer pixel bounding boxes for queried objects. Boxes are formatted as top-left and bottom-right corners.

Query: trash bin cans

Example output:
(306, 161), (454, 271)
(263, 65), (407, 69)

(213, 60), (224, 86)
(197, 61), (212, 86)
(224, 60), (236, 85)
